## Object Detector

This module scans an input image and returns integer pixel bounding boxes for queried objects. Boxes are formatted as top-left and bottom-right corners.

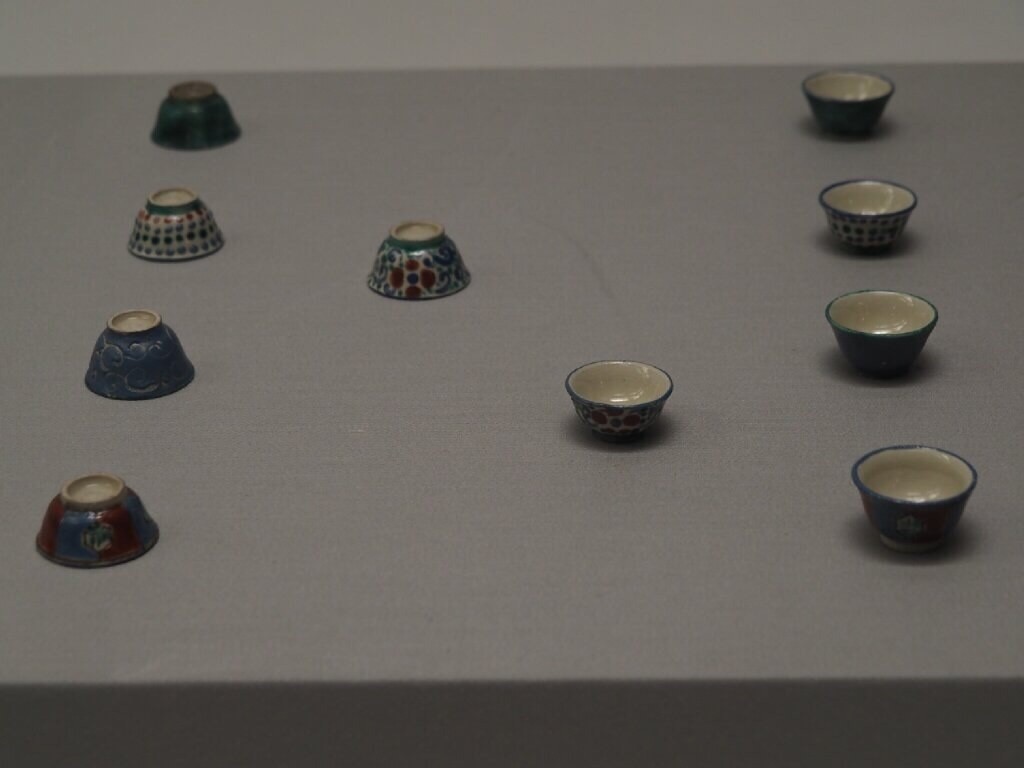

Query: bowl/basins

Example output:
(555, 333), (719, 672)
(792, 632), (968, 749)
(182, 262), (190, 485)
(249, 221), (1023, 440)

(366, 223), (472, 299)
(85, 309), (195, 400)
(126, 187), (225, 261)
(35, 474), (160, 567)
(801, 71), (893, 133)
(850, 443), (979, 557)
(820, 181), (921, 251)
(822, 289), (938, 374)
(566, 360), (674, 442)
(150, 80), (243, 151)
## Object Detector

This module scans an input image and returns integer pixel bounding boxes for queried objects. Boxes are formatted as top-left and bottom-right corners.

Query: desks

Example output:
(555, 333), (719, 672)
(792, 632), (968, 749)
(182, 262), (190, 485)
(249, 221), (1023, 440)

(0, 63), (1024, 767)
